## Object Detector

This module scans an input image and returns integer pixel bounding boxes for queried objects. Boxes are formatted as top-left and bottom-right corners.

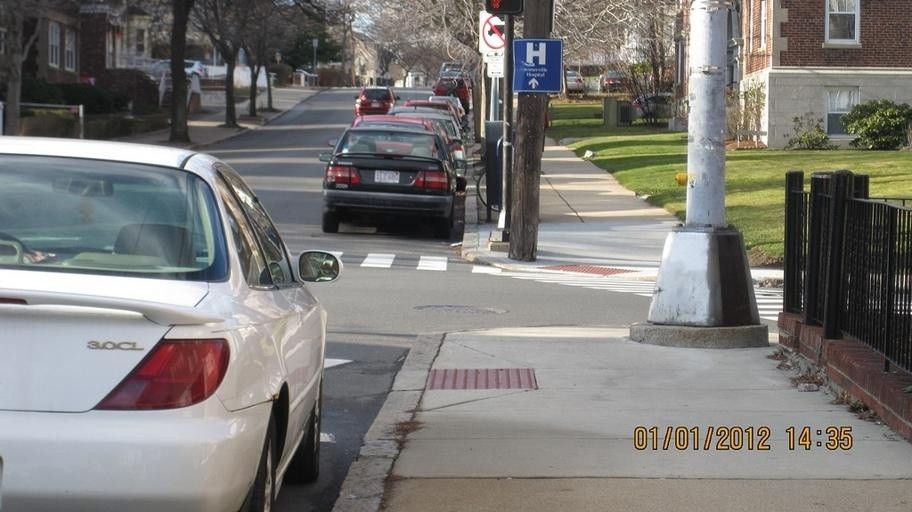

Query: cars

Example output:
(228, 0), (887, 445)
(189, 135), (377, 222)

(566, 71), (586, 92)
(67, 69), (160, 113)
(632, 93), (672, 119)
(1, 135), (343, 512)
(600, 71), (640, 92)
(321, 64), (470, 233)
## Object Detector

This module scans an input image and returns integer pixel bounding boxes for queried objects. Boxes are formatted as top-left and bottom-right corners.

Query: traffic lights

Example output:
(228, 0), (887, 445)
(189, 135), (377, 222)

(485, 1), (523, 15)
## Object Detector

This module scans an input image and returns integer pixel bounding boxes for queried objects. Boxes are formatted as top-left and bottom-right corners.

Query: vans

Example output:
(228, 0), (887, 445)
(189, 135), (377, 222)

(153, 60), (206, 78)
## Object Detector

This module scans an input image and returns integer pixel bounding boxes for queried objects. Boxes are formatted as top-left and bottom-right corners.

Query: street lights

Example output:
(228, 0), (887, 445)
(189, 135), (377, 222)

(311, 38), (319, 87)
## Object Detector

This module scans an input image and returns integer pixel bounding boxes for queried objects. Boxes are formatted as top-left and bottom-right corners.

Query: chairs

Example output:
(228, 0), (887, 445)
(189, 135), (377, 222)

(114, 222), (199, 271)
(348, 137), (432, 158)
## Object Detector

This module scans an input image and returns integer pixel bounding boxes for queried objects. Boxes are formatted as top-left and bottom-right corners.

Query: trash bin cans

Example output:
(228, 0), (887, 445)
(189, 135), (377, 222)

(807, 168), (871, 322)
